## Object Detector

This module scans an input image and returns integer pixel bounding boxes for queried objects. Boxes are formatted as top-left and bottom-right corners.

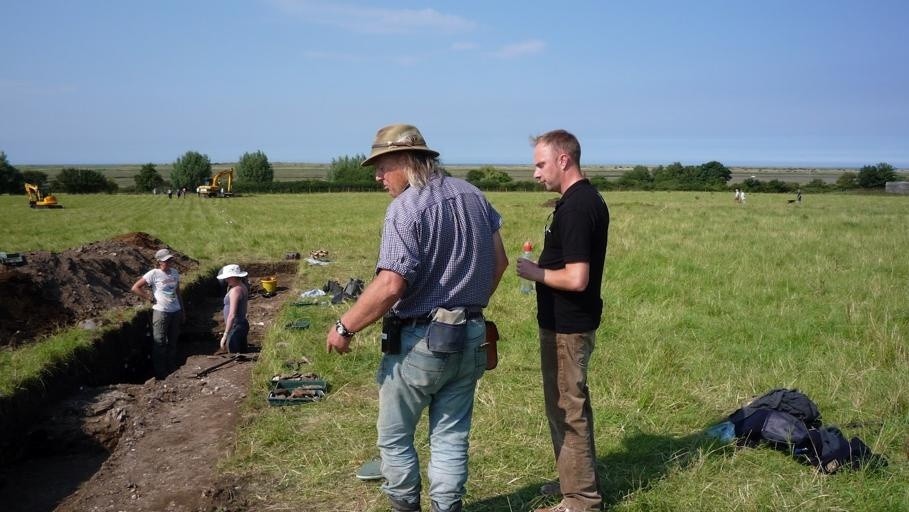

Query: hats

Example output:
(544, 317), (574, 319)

(217, 265), (247, 280)
(155, 248), (174, 262)
(360, 125), (440, 167)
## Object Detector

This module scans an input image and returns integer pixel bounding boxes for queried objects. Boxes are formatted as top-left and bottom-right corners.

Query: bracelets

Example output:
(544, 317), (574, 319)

(223, 332), (228, 336)
(182, 308), (185, 312)
(150, 298), (154, 304)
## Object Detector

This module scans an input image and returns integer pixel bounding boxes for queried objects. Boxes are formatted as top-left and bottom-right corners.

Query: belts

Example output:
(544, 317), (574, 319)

(401, 309), (481, 326)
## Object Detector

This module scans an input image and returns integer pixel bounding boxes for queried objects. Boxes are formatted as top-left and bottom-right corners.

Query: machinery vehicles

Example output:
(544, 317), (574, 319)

(196, 167), (235, 199)
(23, 181), (63, 209)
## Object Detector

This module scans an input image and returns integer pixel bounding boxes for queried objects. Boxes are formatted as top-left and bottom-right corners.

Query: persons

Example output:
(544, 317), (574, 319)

(130, 248), (186, 379)
(322, 122), (507, 512)
(795, 188), (801, 203)
(176, 188), (180, 199)
(513, 129), (612, 512)
(214, 262), (251, 353)
(734, 188), (740, 203)
(181, 188), (186, 199)
(168, 188), (173, 199)
(740, 187), (745, 202)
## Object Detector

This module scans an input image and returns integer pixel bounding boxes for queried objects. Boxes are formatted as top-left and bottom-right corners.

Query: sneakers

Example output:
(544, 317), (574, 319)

(534, 503), (568, 512)
(390, 495), (463, 510)
(541, 484), (563, 498)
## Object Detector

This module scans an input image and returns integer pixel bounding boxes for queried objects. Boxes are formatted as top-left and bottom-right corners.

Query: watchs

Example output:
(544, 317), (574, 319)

(334, 318), (355, 339)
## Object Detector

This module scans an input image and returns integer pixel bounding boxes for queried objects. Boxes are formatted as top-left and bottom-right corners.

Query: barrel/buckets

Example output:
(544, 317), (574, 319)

(259, 277), (278, 293)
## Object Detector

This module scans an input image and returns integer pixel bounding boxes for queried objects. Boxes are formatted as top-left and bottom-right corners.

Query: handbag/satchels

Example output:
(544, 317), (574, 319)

(325, 278), (363, 303)
(706, 390), (880, 473)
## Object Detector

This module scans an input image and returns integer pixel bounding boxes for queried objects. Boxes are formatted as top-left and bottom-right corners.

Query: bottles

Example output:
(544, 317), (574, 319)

(518, 241), (535, 293)
